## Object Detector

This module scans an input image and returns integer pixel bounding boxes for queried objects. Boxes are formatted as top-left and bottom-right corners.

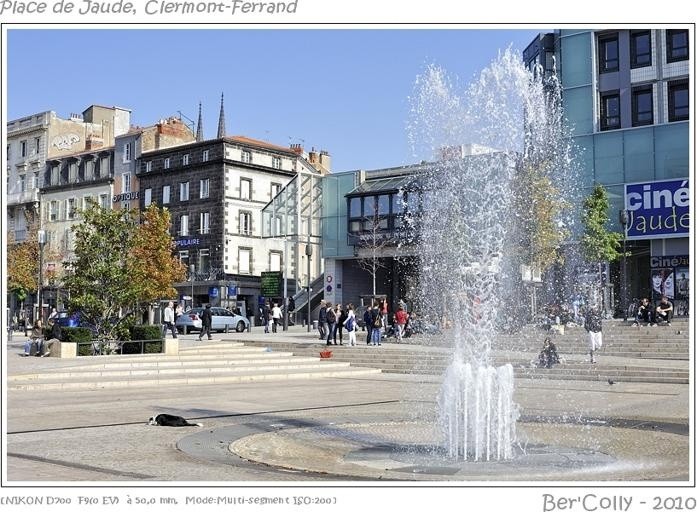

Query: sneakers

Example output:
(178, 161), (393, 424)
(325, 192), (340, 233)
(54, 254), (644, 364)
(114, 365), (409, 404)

(632, 323), (672, 327)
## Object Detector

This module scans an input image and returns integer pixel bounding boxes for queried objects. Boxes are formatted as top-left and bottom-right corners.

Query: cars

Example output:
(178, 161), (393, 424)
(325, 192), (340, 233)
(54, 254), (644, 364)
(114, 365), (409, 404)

(174, 306), (250, 334)
(49, 311), (80, 327)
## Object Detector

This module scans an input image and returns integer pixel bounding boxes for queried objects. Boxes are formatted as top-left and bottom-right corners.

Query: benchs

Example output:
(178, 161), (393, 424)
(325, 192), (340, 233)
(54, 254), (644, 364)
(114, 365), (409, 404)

(162, 336), (179, 354)
(30, 340), (77, 358)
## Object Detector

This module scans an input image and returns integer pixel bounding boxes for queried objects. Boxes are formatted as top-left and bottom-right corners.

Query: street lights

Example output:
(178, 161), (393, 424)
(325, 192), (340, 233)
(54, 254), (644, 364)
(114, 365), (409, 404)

(618, 208), (631, 321)
(305, 244), (313, 333)
(35, 229), (47, 323)
(189, 262), (195, 307)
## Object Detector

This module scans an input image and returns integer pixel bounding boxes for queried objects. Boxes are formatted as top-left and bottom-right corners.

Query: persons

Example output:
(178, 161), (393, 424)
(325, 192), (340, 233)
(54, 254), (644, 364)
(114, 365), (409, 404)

(30, 319), (45, 355)
(679, 272), (689, 296)
(47, 307), (59, 322)
(663, 269), (674, 299)
(40, 318), (62, 357)
(652, 269), (662, 298)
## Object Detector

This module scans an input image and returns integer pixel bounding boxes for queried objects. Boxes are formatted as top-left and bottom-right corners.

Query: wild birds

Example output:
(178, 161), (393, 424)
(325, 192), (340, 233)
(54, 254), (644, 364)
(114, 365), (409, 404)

(607, 378), (614, 384)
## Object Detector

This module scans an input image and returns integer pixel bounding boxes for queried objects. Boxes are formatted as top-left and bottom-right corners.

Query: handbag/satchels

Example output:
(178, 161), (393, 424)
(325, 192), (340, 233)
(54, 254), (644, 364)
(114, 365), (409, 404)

(345, 316), (354, 331)
(374, 320), (381, 328)
(326, 308), (336, 324)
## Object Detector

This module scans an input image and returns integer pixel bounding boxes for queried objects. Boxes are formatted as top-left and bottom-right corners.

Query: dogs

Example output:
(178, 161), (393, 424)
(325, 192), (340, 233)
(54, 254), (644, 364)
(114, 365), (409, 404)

(146, 413), (204, 428)
